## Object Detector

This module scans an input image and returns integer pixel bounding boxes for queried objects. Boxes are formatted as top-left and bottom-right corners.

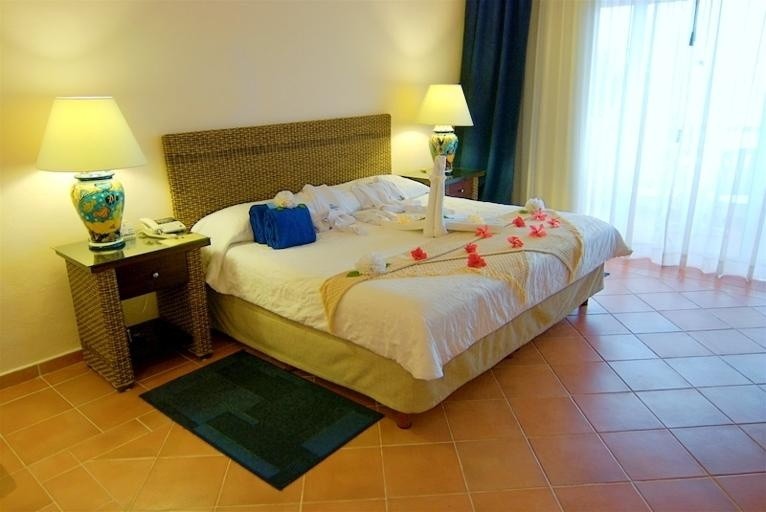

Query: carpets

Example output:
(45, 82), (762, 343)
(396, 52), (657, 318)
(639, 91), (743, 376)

(137, 348), (386, 493)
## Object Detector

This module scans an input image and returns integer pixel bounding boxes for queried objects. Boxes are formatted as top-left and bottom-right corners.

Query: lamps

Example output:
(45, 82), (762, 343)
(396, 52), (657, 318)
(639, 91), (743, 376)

(415, 84), (475, 176)
(33, 95), (150, 254)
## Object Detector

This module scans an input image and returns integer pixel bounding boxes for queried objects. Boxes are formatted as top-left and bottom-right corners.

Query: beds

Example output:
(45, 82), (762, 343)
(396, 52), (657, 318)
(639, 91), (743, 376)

(160, 112), (633, 430)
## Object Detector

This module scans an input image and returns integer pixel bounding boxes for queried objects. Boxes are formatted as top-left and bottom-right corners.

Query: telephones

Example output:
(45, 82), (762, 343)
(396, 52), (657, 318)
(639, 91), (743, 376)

(136, 217), (187, 239)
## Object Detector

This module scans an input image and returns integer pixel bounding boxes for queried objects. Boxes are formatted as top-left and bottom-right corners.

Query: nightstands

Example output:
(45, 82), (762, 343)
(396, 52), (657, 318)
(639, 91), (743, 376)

(398, 167), (486, 202)
(52, 230), (214, 393)
